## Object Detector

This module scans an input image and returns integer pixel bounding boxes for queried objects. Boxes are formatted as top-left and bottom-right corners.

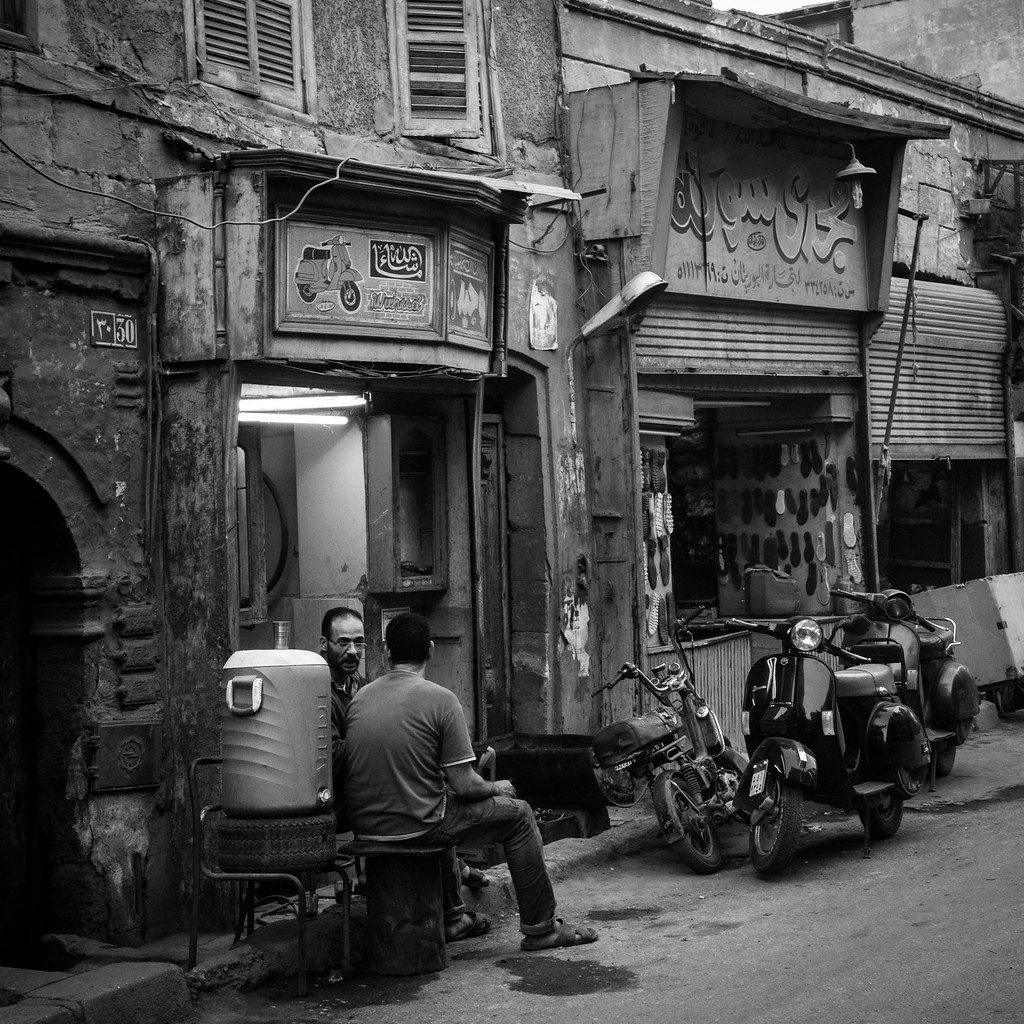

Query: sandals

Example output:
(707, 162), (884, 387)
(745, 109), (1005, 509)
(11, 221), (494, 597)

(354, 870), (368, 896)
(457, 857), (489, 887)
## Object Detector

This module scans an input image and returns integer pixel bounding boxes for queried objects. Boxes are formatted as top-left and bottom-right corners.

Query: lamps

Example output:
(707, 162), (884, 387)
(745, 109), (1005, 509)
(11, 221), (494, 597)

(835, 141), (878, 211)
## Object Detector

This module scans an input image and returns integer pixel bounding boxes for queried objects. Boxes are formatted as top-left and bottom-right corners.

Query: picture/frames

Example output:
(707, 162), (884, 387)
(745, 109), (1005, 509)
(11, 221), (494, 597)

(445, 221), (494, 352)
(271, 203), (446, 343)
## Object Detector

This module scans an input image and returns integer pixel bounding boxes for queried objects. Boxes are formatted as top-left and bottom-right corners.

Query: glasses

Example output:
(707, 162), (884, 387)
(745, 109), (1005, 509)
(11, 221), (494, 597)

(328, 639), (367, 653)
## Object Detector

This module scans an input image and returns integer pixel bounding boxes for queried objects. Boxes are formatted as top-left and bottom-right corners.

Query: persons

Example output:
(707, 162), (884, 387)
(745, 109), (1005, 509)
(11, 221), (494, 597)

(347, 613), (598, 951)
(320, 607), (490, 889)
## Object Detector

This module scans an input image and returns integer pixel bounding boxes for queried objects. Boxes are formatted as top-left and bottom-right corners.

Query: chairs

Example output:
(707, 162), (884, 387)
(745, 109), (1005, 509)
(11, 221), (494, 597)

(187, 754), (356, 997)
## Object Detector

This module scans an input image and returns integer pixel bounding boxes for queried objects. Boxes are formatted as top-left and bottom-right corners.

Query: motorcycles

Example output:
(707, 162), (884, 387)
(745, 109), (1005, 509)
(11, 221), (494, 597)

(826, 587), (981, 776)
(725, 614), (931, 873)
(590, 602), (751, 875)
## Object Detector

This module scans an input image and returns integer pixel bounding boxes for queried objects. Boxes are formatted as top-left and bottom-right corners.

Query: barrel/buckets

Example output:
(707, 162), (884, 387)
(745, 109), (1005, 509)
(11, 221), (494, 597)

(217, 648), (335, 818)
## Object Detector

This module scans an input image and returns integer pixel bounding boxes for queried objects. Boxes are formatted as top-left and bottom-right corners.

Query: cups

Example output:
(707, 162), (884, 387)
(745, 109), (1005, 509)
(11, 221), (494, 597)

(272, 620), (292, 649)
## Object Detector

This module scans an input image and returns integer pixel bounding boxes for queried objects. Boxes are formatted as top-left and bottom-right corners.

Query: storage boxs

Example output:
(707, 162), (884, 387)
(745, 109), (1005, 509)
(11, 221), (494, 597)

(534, 812), (577, 845)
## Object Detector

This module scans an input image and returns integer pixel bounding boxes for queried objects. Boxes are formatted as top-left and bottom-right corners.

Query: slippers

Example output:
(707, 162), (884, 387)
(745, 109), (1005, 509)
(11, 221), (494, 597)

(445, 910), (492, 942)
(522, 918), (598, 951)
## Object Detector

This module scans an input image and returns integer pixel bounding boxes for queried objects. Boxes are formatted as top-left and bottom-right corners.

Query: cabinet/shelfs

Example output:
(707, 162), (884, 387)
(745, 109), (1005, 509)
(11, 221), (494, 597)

(364, 412), (451, 593)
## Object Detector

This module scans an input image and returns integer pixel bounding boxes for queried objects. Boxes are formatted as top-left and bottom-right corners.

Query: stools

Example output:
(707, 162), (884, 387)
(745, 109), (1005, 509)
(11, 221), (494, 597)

(337, 841), (453, 977)
